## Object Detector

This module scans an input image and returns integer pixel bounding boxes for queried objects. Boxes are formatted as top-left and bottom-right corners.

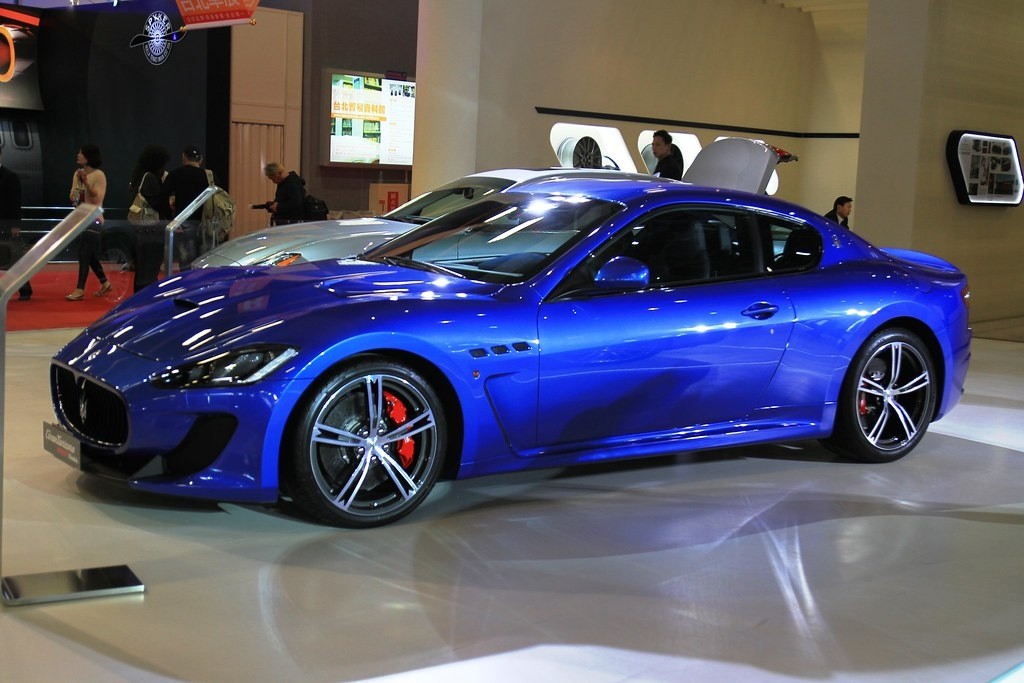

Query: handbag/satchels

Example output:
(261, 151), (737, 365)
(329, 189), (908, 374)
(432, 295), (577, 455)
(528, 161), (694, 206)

(301, 194), (327, 221)
(127, 172), (161, 225)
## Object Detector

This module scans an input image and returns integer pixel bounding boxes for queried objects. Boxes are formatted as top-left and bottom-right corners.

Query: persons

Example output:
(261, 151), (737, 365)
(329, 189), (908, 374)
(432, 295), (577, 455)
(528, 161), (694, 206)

(0, 137), (32, 301)
(122, 145), (175, 295)
(652, 129), (684, 182)
(64, 144), (113, 300)
(163, 144), (222, 271)
(823, 196), (854, 230)
(263, 162), (306, 227)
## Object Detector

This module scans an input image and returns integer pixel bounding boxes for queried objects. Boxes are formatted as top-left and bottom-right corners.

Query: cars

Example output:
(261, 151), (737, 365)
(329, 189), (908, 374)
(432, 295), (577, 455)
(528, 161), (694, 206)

(191, 160), (694, 273)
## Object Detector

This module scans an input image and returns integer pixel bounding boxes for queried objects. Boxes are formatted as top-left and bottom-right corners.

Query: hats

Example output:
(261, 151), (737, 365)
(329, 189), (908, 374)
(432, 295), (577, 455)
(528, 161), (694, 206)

(184, 146), (201, 159)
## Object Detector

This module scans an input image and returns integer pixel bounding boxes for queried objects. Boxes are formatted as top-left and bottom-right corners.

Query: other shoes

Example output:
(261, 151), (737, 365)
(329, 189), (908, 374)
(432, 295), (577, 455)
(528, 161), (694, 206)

(65, 293), (85, 300)
(95, 282), (112, 296)
(18, 296), (31, 301)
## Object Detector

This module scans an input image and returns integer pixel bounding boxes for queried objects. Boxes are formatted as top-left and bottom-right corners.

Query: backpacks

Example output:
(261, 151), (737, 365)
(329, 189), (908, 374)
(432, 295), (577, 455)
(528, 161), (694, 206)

(202, 169), (237, 242)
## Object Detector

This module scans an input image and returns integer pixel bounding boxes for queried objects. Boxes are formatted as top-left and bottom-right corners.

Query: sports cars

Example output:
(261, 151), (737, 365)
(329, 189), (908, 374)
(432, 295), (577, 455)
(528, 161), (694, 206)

(50, 134), (972, 529)
